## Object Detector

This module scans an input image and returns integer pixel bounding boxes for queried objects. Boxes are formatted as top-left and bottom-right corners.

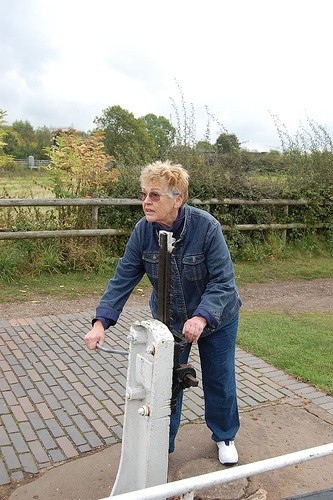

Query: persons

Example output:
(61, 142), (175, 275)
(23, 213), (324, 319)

(82, 158), (243, 466)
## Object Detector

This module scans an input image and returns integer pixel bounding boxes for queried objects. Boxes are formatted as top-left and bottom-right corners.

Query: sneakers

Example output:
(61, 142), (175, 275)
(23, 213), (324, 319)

(216, 441), (238, 465)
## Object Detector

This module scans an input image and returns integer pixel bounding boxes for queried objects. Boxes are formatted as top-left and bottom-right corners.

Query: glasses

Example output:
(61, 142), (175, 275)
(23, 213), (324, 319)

(138, 191), (179, 202)
